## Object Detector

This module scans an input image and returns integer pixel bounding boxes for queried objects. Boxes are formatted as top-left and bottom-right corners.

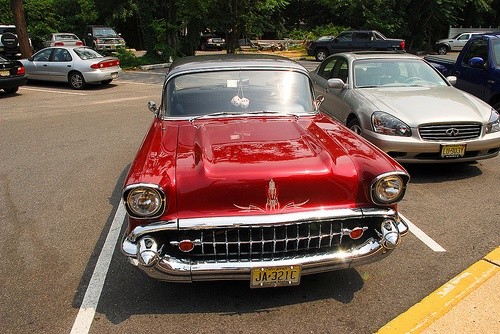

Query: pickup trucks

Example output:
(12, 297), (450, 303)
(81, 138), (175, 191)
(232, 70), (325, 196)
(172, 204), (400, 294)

(423, 31), (500, 113)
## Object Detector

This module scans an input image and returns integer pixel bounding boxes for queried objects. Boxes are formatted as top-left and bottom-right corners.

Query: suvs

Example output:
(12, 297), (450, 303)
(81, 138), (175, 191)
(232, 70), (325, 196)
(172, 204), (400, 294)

(434, 30), (488, 55)
(0, 24), (34, 60)
(82, 24), (125, 54)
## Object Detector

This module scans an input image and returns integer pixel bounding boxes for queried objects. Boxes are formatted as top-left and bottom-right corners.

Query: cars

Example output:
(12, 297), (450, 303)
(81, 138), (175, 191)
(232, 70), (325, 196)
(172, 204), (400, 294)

(14, 46), (123, 90)
(42, 33), (83, 48)
(198, 28), (227, 51)
(308, 49), (500, 166)
(307, 29), (407, 63)
(0, 53), (26, 95)
(120, 53), (411, 289)
